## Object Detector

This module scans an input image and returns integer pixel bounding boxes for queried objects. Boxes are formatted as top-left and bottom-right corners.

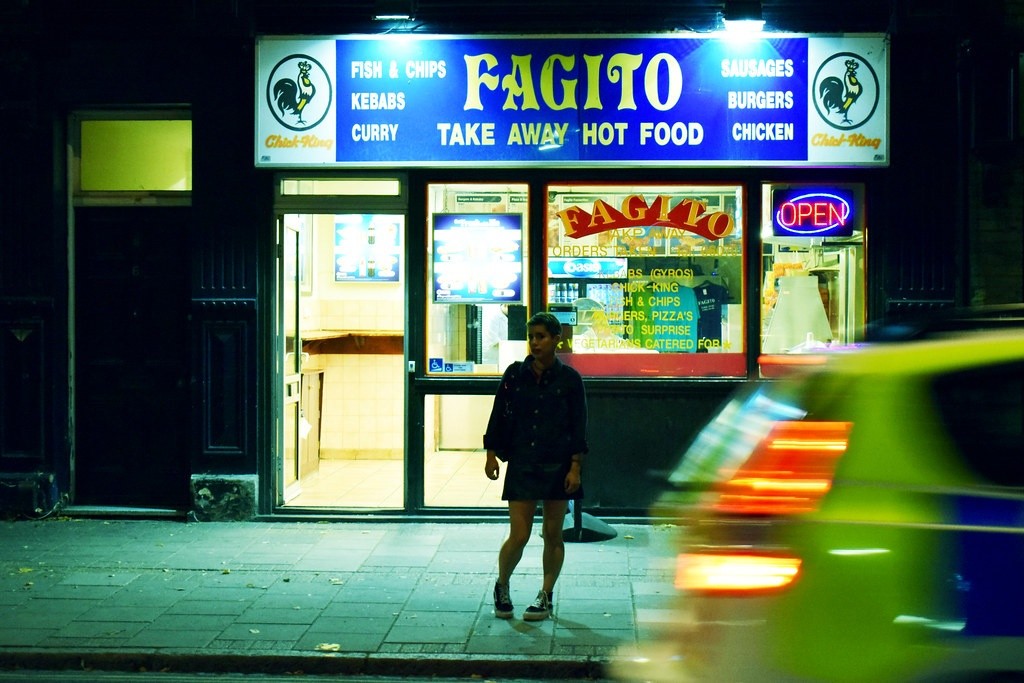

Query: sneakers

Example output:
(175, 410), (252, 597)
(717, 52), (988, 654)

(494, 581), (514, 618)
(523, 591), (553, 620)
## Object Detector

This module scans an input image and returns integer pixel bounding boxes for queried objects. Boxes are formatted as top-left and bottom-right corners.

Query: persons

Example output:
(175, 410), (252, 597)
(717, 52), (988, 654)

(484, 312), (590, 619)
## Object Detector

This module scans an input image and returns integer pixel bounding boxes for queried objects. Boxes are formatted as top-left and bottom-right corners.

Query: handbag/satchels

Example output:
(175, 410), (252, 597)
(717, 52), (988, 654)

(495, 362), (521, 462)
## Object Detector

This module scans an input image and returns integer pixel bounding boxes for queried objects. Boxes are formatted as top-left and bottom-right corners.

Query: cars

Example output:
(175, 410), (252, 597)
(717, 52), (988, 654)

(595, 328), (1024, 683)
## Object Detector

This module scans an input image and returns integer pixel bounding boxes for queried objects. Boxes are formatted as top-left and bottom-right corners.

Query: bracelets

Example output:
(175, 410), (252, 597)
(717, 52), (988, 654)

(572, 459), (582, 463)
